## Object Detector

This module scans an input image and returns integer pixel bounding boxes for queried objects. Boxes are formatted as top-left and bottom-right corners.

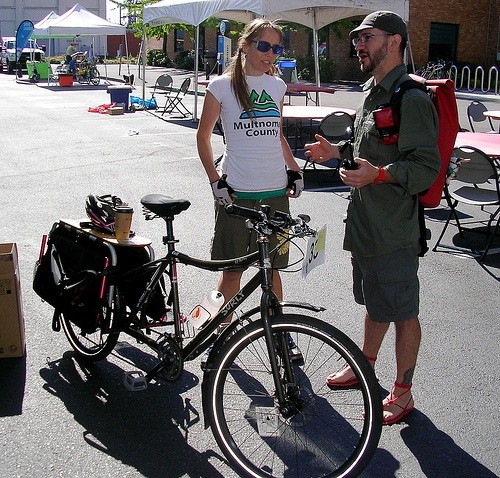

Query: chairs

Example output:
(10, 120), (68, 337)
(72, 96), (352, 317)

(466, 100), (500, 169)
(302, 110), (356, 177)
(431, 145), (500, 265)
(148, 73), (174, 113)
(161, 77), (194, 118)
(49, 65), (68, 86)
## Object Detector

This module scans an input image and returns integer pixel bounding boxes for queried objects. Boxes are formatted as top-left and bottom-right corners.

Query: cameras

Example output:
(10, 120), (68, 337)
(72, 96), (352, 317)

(340, 158), (359, 170)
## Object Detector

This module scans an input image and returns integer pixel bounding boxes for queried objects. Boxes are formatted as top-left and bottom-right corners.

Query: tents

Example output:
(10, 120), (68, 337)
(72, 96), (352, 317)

(30, 3), (130, 74)
(143, 0), (409, 107)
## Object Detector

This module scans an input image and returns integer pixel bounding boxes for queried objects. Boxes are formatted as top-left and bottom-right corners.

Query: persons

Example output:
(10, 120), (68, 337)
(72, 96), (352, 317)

(304, 10), (442, 424)
(196, 18), (302, 366)
(66, 42), (76, 62)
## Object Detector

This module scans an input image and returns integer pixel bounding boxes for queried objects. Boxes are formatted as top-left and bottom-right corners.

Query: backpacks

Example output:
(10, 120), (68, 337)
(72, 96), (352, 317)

(373, 73), (460, 209)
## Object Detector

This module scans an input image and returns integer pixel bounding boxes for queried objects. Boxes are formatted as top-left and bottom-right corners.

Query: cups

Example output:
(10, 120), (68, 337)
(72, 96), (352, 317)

(113, 206), (134, 242)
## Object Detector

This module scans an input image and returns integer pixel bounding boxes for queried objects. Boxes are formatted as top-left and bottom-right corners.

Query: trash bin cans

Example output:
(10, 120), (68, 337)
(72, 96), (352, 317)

(278, 68), (292, 83)
(58, 74), (74, 87)
(107, 86), (132, 112)
(204, 55), (216, 72)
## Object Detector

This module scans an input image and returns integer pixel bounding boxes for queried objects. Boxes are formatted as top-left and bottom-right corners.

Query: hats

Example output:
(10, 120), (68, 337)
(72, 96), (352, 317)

(349, 11), (409, 41)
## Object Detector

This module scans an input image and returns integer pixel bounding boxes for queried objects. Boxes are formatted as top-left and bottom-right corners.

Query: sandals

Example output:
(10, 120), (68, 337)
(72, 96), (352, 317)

(327, 354), (377, 387)
(362, 382), (414, 424)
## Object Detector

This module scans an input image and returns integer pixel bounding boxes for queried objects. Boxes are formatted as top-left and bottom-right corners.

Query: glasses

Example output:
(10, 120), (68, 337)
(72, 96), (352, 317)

(352, 33), (398, 47)
(249, 38), (284, 55)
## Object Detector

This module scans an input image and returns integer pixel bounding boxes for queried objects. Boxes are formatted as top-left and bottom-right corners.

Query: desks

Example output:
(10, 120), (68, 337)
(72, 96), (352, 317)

(482, 109), (500, 162)
(440, 131), (500, 223)
(281, 105), (357, 172)
(284, 83), (338, 131)
(193, 79), (211, 89)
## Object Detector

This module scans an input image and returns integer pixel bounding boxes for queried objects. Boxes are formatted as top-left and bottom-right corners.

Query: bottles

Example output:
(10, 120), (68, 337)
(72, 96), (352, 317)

(188, 291), (225, 331)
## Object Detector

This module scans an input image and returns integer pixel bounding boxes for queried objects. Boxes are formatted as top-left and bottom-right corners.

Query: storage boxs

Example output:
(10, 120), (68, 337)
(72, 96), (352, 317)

(0, 240), (27, 359)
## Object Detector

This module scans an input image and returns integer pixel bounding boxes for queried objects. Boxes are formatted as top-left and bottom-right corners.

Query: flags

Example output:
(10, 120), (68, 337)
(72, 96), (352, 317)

(16, 20), (34, 62)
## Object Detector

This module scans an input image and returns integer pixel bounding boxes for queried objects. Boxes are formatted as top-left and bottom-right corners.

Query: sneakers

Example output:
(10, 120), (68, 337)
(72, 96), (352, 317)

(200, 338), (231, 371)
(274, 329), (302, 360)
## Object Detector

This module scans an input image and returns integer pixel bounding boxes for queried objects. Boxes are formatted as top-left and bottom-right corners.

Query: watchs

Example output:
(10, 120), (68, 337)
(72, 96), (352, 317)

(374, 168), (387, 185)
